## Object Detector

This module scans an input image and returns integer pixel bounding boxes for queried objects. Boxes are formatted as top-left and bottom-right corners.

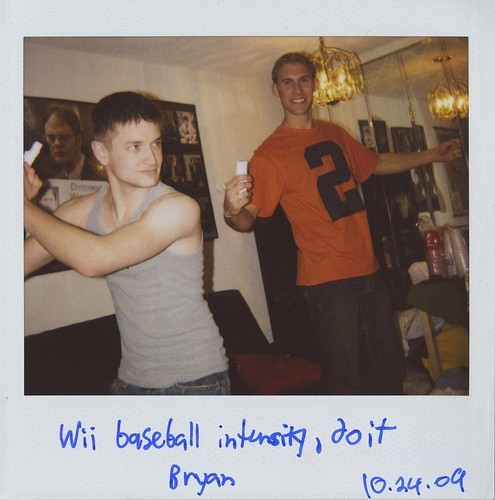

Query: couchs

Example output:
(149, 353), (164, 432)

(24, 288), (276, 395)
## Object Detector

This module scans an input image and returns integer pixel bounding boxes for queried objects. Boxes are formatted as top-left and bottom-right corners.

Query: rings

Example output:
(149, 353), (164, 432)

(239, 193), (245, 199)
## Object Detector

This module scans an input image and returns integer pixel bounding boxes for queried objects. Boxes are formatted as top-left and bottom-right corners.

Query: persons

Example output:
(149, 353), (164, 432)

(24, 92), (233, 395)
(177, 115), (197, 144)
(189, 158), (200, 181)
(37, 188), (57, 209)
(44, 107), (98, 181)
(223, 52), (464, 396)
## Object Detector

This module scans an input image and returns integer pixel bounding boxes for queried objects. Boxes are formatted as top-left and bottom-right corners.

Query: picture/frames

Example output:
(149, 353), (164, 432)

(147, 99), (219, 241)
(390, 125), (440, 214)
(357, 120), (398, 208)
(23, 95), (109, 276)
(433, 125), (468, 217)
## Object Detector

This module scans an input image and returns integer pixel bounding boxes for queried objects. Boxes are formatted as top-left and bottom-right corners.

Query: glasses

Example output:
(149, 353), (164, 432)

(43, 132), (76, 144)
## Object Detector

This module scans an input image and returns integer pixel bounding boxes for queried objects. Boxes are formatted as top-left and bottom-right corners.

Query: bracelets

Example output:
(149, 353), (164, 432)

(223, 210), (242, 219)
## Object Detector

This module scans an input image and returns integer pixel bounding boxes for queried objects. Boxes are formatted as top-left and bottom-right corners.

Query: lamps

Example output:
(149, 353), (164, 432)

(307, 36), (368, 107)
(426, 37), (469, 122)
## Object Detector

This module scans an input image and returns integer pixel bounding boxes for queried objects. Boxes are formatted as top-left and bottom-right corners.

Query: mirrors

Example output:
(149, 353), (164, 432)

(327, 37), (468, 243)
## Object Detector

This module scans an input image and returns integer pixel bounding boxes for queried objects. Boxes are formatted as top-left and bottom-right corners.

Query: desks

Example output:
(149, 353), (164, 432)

(408, 276), (468, 382)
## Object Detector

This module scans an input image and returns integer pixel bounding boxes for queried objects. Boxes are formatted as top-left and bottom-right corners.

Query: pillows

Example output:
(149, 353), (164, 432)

(226, 349), (322, 395)
